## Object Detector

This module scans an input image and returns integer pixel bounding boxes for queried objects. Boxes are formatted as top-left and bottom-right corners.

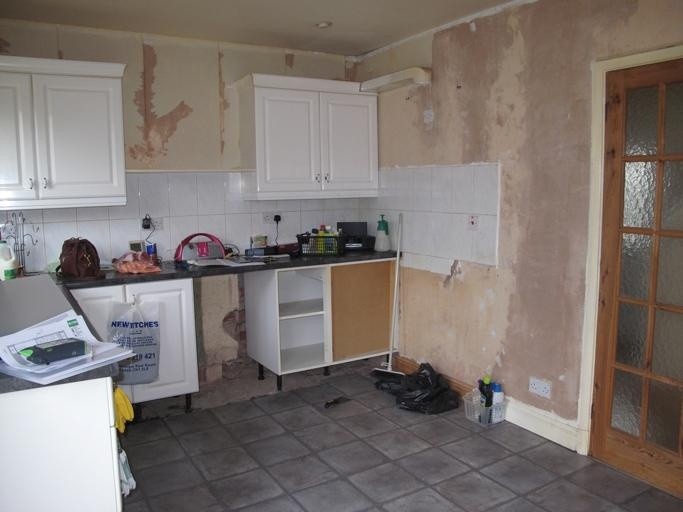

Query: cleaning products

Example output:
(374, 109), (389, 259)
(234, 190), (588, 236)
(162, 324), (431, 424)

(0, 240), (19, 281)
(374, 214), (391, 252)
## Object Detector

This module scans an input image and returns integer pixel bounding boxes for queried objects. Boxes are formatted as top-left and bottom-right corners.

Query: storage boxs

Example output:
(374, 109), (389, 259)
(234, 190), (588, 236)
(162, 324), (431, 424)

(463, 392), (510, 427)
(299, 233), (342, 256)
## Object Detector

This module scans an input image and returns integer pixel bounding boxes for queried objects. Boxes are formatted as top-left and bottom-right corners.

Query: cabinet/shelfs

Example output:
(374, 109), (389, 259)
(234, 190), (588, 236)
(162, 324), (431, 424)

(32, 58), (126, 209)
(124, 277), (200, 413)
(0, 272), (123, 512)
(320, 80), (377, 198)
(234, 71), (322, 201)
(244, 264), (330, 393)
(70, 280), (133, 406)
(0, 57), (41, 210)
(330, 258), (399, 369)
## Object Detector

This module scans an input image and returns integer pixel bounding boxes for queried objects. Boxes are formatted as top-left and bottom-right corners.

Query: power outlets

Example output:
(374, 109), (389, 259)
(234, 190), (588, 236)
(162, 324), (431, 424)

(263, 211), (282, 225)
(529, 377), (552, 400)
(142, 217), (163, 231)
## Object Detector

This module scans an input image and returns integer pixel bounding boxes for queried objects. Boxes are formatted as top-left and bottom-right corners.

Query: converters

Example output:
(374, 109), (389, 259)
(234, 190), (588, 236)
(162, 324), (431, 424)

(142, 216), (151, 230)
